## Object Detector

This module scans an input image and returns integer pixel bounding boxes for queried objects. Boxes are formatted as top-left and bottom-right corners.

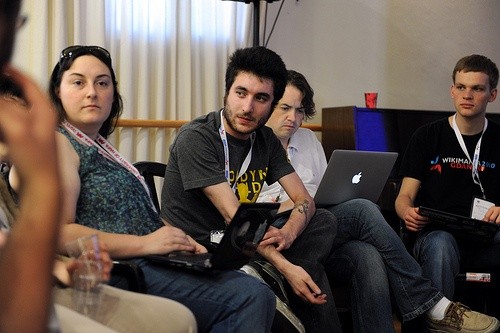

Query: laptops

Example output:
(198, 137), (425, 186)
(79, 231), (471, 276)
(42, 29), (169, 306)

(142, 202), (281, 281)
(313, 150), (398, 208)
(419, 206), (500, 242)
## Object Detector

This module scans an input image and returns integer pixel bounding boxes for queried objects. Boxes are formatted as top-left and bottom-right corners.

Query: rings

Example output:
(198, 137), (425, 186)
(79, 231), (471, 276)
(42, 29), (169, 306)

(183, 233), (186, 239)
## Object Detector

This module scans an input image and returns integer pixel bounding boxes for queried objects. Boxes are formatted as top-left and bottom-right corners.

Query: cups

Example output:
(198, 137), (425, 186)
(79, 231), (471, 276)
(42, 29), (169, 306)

(365, 92), (377, 108)
(73, 277), (104, 319)
(63, 234), (102, 277)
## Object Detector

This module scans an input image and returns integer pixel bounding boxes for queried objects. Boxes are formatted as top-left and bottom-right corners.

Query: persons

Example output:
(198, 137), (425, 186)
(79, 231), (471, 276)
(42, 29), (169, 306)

(394, 54), (500, 314)
(256, 69), (500, 333)
(161, 48), (336, 333)
(46, 45), (277, 332)
(1, 0), (197, 332)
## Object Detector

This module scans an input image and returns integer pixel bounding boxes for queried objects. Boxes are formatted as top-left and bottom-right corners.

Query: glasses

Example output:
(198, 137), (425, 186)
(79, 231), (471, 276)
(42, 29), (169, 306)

(60, 44), (110, 67)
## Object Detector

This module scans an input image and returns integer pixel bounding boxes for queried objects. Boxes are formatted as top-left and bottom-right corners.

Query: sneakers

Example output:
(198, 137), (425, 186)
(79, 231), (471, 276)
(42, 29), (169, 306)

(429, 300), (500, 333)
